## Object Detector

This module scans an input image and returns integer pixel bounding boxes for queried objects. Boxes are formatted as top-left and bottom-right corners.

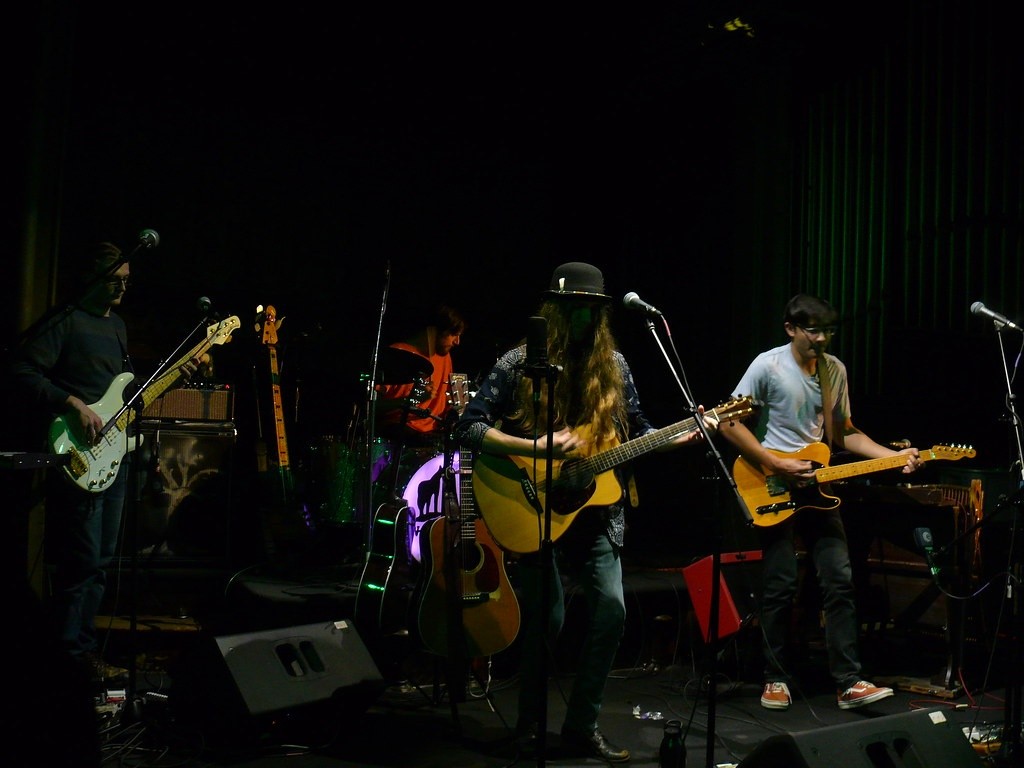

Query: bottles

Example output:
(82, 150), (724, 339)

(658, 719), (687, 768)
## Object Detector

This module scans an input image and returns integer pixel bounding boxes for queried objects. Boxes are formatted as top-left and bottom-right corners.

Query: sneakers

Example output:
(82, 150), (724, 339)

(837, 680), (895, 710)
(559, 724), (631, 763)
(514, 706), (546, 759)
(761, 681), (793, 709)
(69, 652), (129, 684)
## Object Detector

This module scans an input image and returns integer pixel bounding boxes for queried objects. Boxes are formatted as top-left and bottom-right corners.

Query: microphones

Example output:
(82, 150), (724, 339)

(623, 291), (662, 315)
(198, 296), (221, 322)
(526, 315), (549, 416)
(913, 527), (940, 586)
(140, 229), (159, 248)
(971, 302), (1022, 333)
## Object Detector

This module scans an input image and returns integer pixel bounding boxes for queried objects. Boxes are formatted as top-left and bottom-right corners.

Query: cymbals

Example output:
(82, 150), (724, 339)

(349, 345), (435, 386)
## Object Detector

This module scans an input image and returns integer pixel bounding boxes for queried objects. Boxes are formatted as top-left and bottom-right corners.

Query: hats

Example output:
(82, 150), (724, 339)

(543, 261), (613, 301)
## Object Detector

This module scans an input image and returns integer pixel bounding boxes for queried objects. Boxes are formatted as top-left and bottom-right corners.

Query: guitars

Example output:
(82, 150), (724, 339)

(470, 393), (756, 555)
(49, 312), (244, 494)
(731, 442), (977, 527)
(354, 376), (431, 644)
(254, 302), (322, 581)
(409, 372), (522, 659)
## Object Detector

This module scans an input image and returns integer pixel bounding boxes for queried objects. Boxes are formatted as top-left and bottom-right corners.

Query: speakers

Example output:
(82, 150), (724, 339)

(736, 706), (985, 768)
(172, 617), (380, 738)
(682, 550), (765, 643)
(110, 418), (236, 568)
(849, 486), (984, 642)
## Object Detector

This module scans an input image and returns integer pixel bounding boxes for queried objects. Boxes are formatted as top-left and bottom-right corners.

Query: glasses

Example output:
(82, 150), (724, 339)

(96, 274), (133, 286)
(795, 322), (839, 338)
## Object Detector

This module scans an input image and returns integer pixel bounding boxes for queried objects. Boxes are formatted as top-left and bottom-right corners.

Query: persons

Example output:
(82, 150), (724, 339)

(719, 294), (922, 710)
(15, 243), (201, 681)
(455, 262), (719, 762)
(376, 304), (464, 432)
